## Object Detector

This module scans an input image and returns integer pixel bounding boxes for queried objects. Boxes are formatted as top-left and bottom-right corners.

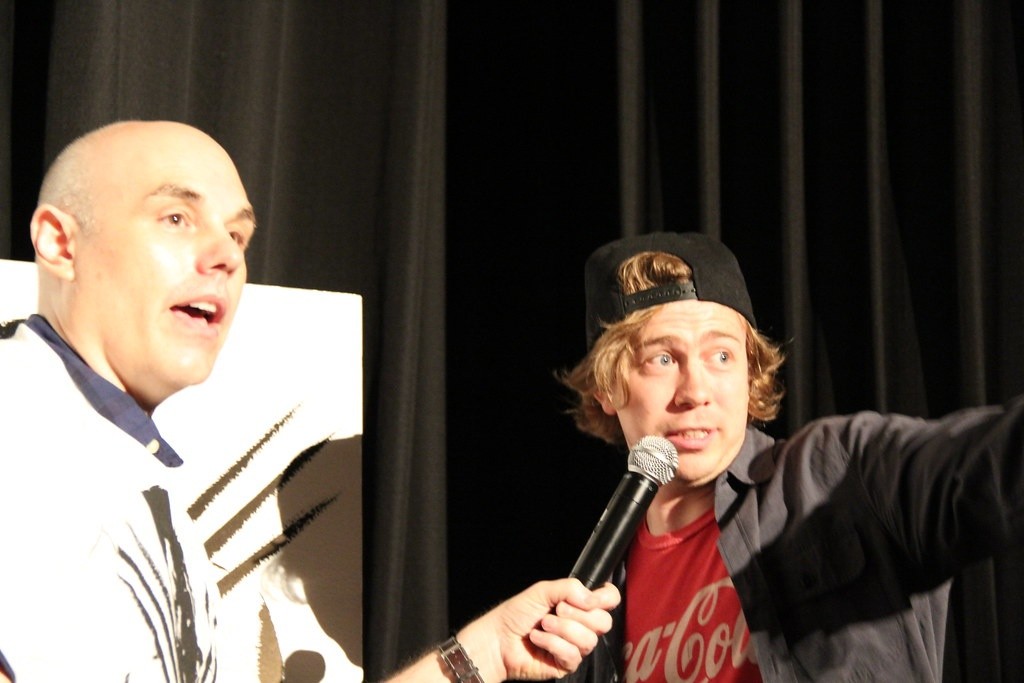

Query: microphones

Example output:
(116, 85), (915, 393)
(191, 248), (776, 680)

(565, 435), (679, 589)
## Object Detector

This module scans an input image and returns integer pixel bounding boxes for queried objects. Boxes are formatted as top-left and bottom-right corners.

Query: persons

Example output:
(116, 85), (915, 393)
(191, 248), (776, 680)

(561, 231), (1023, 683)
(1, 120), (622, 683)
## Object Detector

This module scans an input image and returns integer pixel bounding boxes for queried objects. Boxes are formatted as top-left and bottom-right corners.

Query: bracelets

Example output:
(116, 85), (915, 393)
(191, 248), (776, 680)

(438, 637), (485, 683)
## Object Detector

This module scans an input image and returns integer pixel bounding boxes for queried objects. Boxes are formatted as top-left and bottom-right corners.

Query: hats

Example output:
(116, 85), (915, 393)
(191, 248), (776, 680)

(581, 232), (758, 356)
